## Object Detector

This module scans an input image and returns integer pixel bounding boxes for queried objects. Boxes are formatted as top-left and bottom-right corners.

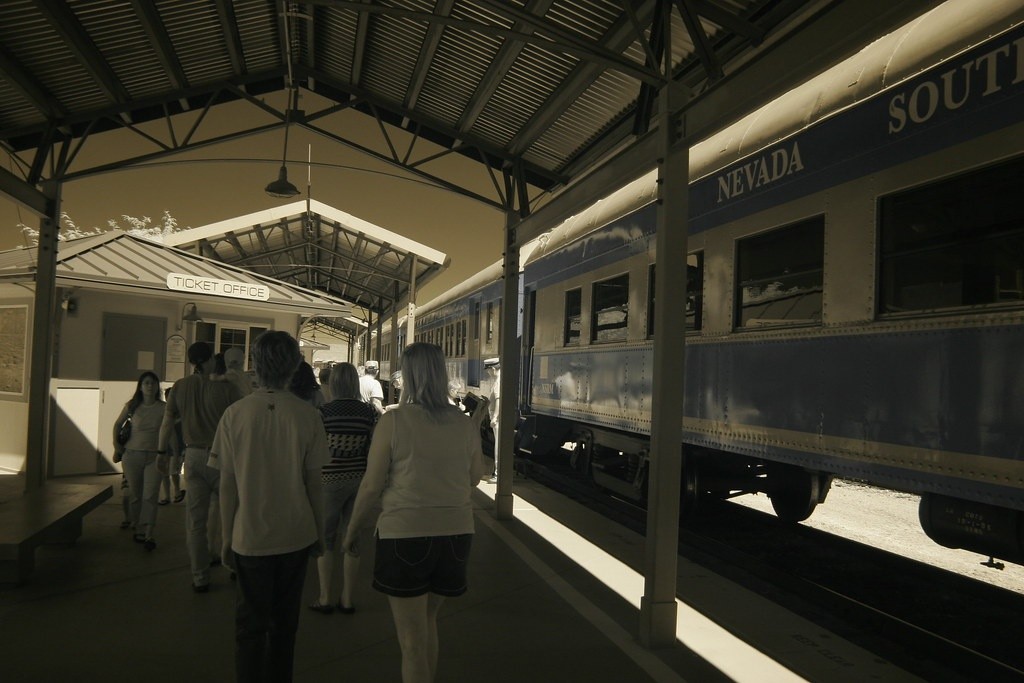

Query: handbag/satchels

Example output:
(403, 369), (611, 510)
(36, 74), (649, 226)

(117, 400), (134, 445)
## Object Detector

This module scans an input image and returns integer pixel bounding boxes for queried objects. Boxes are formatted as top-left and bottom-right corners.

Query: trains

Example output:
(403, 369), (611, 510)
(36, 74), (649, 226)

(355, 0), (1023, 572)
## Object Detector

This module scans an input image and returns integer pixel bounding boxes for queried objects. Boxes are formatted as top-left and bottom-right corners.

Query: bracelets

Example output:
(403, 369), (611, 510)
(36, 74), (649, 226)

(157, 450), (167, 454)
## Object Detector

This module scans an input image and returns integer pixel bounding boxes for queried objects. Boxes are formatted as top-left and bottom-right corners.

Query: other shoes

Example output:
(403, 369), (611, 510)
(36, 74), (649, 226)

(335, 598), (356, 614)
(192, 581), (209, 594)
(488, 475), (497, 483)
(134, 533), (145, 543)
(308, 599), (332, 614)
(208, 554), (221, 566)
(120, 521), (132, 529)
(144, 541), (156, 550)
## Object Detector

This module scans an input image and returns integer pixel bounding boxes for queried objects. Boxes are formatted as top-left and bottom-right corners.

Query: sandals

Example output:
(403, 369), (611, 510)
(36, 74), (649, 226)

(158, 498), (170, 505)
(173, 490), (186, 503)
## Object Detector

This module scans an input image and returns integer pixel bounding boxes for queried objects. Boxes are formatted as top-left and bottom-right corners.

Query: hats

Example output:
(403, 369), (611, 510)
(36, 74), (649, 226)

(188, 341), (211, 365)
(224, 347), (244, 368)
(390, 371), (402, 385)
(483, 358), (499, 370)
(365, 361), (378, 369)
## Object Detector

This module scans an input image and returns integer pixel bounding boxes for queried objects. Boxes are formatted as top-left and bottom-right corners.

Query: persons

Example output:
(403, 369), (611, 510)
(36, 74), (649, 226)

(554, 363), (588, 419)
(156, 343), (469, 614)
(207, 332), (331, 683)
(342, 343), (490, 683)
(112, 371), (186, 550)
(484, 357), (500, 483)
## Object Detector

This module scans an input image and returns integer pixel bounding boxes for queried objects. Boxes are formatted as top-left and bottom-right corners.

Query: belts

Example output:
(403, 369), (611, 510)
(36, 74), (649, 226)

(183, 443), (212, 450)
